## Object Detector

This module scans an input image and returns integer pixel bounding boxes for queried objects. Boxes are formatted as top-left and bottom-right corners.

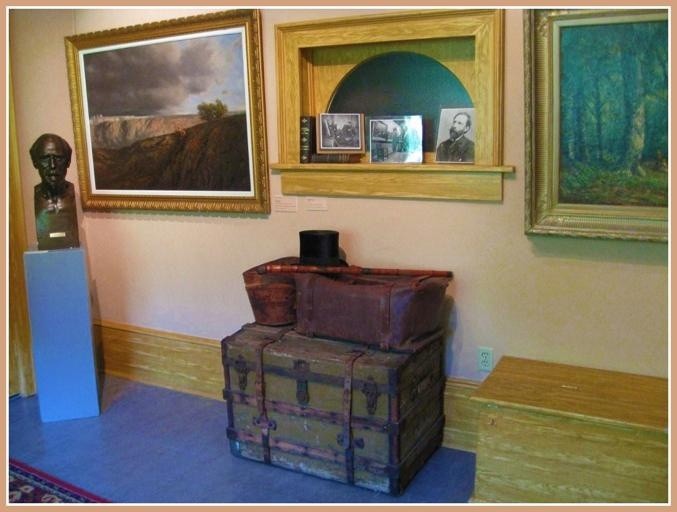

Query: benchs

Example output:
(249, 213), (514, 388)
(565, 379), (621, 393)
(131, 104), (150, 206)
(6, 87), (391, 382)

(465, 354), (669, 504)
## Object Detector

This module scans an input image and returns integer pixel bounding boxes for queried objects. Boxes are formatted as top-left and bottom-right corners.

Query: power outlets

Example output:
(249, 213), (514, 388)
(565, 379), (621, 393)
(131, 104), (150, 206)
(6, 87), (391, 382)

(475, 343), (496, 374)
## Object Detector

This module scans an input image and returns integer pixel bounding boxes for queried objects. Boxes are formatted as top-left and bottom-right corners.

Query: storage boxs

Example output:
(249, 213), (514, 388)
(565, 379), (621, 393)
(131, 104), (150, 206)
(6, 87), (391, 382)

(219, 322), (448, 499)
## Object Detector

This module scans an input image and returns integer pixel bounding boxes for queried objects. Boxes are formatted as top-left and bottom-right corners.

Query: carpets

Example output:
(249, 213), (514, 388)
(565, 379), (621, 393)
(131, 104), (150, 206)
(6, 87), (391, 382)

(8, 456), (112, 503)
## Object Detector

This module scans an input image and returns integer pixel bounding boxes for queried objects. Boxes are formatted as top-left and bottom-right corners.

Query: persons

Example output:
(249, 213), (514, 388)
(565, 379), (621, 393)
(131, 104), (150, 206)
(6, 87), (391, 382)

(27, 133), (82, 251)
(390, 126), (417, 154)
(435, 111), (474, 163)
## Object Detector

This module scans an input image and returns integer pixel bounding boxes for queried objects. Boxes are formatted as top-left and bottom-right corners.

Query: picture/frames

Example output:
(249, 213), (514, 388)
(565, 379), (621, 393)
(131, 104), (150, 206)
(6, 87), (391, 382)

(364, 113), (424, 164)
(512, 8), (669, 249)
(315, 111), (367, 156)
(57, 5), (273, 222)
